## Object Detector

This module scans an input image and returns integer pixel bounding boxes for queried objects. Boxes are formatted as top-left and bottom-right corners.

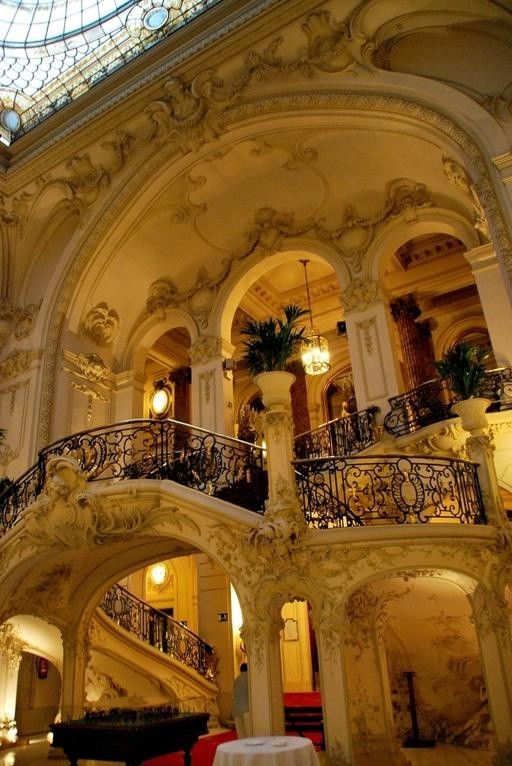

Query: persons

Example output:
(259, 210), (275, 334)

(230, 663), (252, 740)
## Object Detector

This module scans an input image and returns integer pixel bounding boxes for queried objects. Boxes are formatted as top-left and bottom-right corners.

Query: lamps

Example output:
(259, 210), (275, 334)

(297, 256), (332, 377)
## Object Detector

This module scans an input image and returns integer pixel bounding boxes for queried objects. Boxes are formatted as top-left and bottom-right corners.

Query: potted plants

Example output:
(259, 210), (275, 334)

(234, 304), (313, 411)
(429, 338), (502, 435)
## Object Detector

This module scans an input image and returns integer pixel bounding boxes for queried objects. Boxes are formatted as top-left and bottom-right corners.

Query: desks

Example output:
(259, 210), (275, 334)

(47, 710), (211, 766)
(216, 735), (314, 766)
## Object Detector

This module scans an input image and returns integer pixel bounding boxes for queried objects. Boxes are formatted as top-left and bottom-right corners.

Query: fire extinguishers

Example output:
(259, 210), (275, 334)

(36, 656), (48, 680)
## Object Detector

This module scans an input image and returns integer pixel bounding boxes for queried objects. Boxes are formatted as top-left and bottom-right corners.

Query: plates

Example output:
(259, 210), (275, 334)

(245, 740), (265, 746)
